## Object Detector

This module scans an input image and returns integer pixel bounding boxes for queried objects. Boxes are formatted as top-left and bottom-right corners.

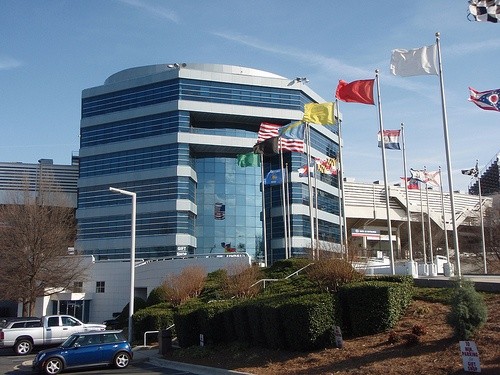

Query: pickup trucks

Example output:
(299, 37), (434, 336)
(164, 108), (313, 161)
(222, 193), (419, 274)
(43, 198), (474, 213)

(0, 314), (106, 355)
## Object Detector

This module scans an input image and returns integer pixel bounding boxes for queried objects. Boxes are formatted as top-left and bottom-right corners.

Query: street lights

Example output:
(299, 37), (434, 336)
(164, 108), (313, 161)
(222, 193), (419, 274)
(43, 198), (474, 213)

(108, 188), (136, 342)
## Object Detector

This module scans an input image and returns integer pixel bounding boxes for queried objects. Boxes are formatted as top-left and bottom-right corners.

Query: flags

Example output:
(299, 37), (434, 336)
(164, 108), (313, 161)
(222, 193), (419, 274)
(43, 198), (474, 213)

(461, 164), (479, 178)
(400, 176), (418, 190)
(409, 168), (426, 183)
(425, 171), (441, 188)
(277, 121), (306, 140)
(303, 102), (336, 127)
(467, 0), (500, 24)
(467, 85), (500, 112)
(334, 79), (376, 106)
(265, 169), (283, 185)
(314, 157), (338, 175)
(297, 164), (314, 178)
(377, 128), (402, 150)
(389, 42), (439, 76)
(257, 121), (304, 154)
(236, 152), (260, 168)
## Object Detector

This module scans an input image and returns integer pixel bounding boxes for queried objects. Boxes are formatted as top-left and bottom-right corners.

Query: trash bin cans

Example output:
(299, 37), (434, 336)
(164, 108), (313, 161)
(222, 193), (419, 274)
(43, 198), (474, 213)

(158, 330), (172, 354)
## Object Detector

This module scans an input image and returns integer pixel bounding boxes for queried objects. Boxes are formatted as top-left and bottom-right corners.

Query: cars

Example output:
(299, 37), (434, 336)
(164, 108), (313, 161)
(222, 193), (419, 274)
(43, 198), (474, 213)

(0, 317), (42, 328)
(33, 329), (133, 375)
